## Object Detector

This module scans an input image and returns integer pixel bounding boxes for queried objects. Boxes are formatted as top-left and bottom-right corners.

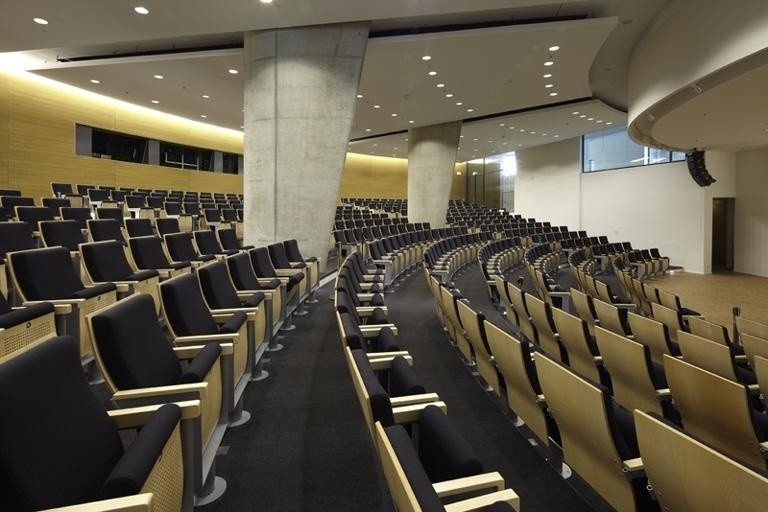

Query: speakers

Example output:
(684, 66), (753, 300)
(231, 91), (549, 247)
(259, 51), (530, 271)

(687, 151), (716, 186)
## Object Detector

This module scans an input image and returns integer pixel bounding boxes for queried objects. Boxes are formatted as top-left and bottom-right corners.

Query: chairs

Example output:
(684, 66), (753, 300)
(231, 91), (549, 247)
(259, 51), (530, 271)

(0, 182), (768, 512)
